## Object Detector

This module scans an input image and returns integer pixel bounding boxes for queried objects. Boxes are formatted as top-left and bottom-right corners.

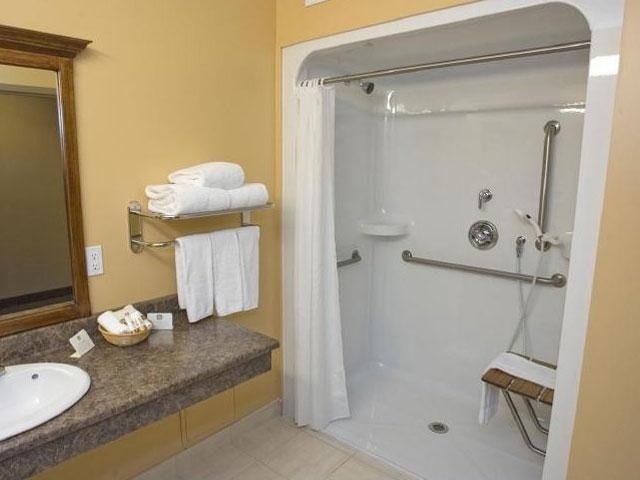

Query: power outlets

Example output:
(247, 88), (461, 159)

(85, 245), (105, 277)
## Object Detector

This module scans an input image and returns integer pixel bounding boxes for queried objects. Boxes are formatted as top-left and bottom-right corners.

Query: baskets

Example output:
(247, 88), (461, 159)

(97, 315), (153, 347)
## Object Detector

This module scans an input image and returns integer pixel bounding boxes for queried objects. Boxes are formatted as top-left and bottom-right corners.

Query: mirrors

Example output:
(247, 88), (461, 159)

(0, 23), (94, 338)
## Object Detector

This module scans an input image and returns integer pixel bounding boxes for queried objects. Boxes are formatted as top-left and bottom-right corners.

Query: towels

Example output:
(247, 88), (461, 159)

(175, 225), (261, 324)
(478, 351), (556, 428)
(144, 160), (269, 216)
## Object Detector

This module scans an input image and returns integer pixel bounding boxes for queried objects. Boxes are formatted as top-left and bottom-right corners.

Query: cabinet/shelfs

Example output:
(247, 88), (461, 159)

(126, 200), (275, 255)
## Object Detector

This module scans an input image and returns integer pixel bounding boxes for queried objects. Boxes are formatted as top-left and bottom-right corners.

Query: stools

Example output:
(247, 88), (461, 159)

(480, 351), (556, 457)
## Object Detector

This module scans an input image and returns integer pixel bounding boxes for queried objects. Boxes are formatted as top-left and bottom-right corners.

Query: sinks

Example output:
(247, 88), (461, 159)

(0, 362), (92, 441)
